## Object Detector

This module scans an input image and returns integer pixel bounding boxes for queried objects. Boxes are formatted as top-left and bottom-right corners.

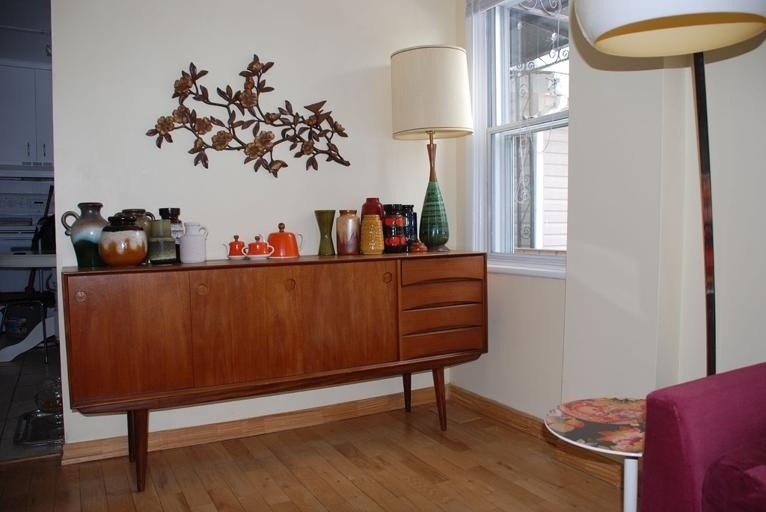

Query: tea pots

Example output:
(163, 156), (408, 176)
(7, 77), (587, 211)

(266, 223), (304, 259)
(242, 236), (275, 260)
(222, 234), (246, 259)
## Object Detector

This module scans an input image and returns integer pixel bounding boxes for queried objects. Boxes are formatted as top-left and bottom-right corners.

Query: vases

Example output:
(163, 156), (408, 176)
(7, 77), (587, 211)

(359, 213), (386, 256)
(335, 209), (361, 256)
(313, 209), (336, 256)
(361, 198), (384, 222)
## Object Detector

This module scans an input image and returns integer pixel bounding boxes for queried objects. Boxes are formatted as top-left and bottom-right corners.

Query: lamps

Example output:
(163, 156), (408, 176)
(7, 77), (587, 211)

(390, 43), (476, 253)
(573, 2), (766, 377)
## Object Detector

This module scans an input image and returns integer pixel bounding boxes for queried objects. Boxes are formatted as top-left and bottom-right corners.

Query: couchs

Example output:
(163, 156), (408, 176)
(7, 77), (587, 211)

(636, 357), (766, 512)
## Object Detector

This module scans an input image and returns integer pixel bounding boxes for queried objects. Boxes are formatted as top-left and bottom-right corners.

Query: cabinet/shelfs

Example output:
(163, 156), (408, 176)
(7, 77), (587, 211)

(61, 248), (489, 493)
